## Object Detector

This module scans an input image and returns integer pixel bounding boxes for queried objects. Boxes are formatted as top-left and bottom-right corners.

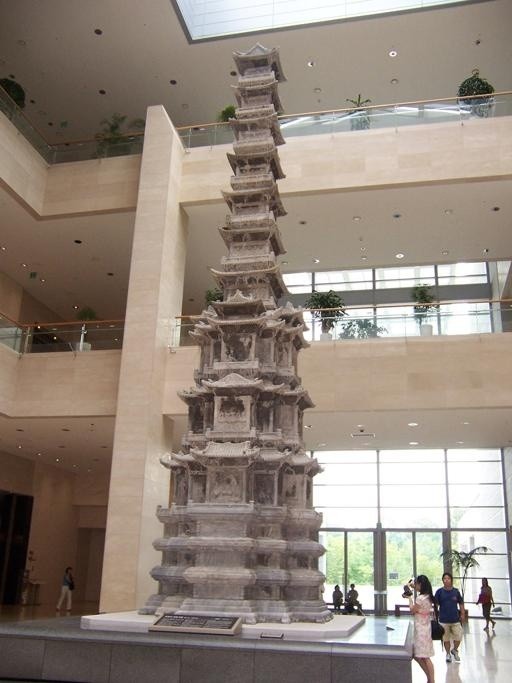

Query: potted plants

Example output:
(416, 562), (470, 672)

(409, 282), (440, 337)
(73, 305), (98, 351)
(90, 110), (147, 159)
(304, 289), (348, 341)
(336, 93), (373, 132)
(456, 67), (498, 120)
(439, 545), (494, 625)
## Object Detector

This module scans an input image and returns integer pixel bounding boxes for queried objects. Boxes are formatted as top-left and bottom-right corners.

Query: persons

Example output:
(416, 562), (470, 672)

(402, 573), (466, 683)
(475, 577), (496, 630)
(55, 566), (75, 612)
(333, 584), (359, 615)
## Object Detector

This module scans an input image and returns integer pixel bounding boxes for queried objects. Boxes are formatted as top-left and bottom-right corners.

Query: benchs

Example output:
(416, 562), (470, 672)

(326, 603), (362, 615)
(395, 604), (438, 618)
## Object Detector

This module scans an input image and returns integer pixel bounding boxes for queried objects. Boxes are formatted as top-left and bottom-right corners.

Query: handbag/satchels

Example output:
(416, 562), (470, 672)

(430, 620), (446, 640)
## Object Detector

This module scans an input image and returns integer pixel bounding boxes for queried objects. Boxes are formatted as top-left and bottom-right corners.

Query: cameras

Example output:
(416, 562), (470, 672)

(402, 585), (412, 598)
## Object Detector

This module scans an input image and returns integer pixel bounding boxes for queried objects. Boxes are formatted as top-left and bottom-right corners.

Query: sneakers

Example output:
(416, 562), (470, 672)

(446, 654), (452, 662)
(492, 622), (496, 628)
(483, 627), (489, 630)
(451, 649), (460, 661)
